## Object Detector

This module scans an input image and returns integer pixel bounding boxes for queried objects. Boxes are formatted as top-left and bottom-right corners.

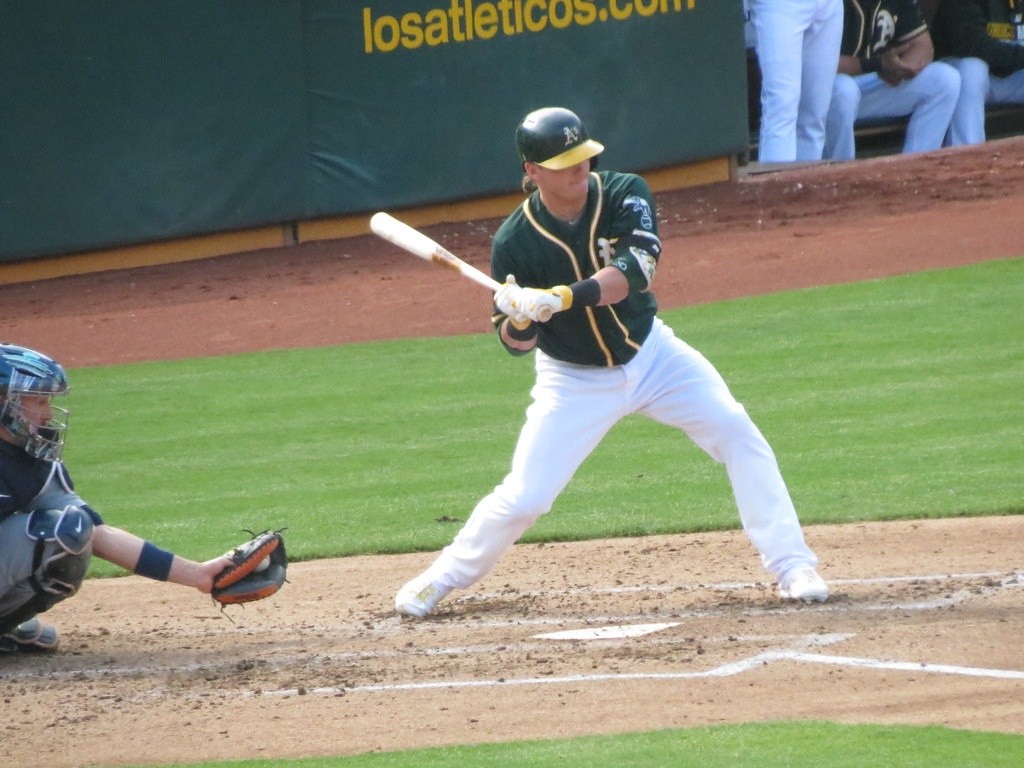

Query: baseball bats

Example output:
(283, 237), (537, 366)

(369, 211), (554, 324)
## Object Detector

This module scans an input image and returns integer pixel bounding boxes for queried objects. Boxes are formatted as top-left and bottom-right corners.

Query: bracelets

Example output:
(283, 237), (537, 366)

(552, 284), (572, 311)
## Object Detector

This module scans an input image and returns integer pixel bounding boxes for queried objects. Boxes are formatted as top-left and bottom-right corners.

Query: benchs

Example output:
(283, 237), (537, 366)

(749, 107), (1024, 151)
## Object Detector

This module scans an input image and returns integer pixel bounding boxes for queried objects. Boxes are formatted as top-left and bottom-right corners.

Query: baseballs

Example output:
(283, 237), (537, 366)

(252, 554), (270, 571)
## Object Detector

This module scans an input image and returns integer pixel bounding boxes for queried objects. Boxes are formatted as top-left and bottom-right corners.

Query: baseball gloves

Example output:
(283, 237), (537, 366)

(208, 531), (290, 605)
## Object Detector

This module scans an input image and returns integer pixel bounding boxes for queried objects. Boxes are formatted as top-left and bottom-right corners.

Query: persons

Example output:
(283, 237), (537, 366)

(744, 0), (1024, 163)
(393, 105), (830, 619)
(0, 342), (288, 653)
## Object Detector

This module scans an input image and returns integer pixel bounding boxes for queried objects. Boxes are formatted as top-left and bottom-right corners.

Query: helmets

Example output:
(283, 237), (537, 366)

(515, 106), (604, 169)
(0, 344), (69, 462)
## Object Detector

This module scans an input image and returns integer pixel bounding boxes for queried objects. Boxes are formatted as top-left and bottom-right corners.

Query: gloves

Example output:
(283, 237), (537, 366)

(493, 274), (539, 331)
(512, 286), (573, 323)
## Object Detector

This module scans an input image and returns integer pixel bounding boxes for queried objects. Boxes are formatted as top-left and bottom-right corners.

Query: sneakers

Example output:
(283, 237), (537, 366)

(394, 570), (447, 616)
(778, 566), (831, 599)
